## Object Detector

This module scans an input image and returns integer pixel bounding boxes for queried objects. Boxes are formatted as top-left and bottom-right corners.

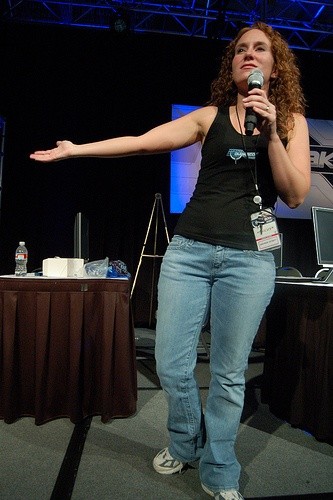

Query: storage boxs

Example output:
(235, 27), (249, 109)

(42, 257), (84, 277)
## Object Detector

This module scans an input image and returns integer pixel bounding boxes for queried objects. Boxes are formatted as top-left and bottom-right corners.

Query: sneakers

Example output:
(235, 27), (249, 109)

(153, 446), (187, 475)
(200, 481), (244, 500)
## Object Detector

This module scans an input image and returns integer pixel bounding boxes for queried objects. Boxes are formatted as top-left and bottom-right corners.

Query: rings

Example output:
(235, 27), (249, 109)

(266, 106), (269, 111)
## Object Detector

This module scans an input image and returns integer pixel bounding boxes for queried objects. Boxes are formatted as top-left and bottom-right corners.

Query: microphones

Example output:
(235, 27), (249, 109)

(243, 68), (264, 137)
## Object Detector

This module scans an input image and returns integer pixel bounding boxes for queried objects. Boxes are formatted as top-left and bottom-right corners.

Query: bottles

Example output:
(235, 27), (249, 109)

(15, 242), (28, 277)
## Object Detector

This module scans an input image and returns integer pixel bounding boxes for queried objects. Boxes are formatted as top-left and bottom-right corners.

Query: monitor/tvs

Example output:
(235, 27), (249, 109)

(270, 233), (284, 268)
(311, 206), (333, 269)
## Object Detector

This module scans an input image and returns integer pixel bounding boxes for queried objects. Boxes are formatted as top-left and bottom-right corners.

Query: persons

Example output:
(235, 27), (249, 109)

(30, 21), (311, 500)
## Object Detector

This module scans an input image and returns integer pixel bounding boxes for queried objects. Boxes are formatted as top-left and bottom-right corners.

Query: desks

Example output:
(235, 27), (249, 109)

(261, 280), (333, 447)
(0, 275), (137, 426)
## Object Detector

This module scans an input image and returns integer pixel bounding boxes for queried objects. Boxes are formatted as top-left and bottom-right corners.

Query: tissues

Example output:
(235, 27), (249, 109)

(42, 255), (84, 277)
